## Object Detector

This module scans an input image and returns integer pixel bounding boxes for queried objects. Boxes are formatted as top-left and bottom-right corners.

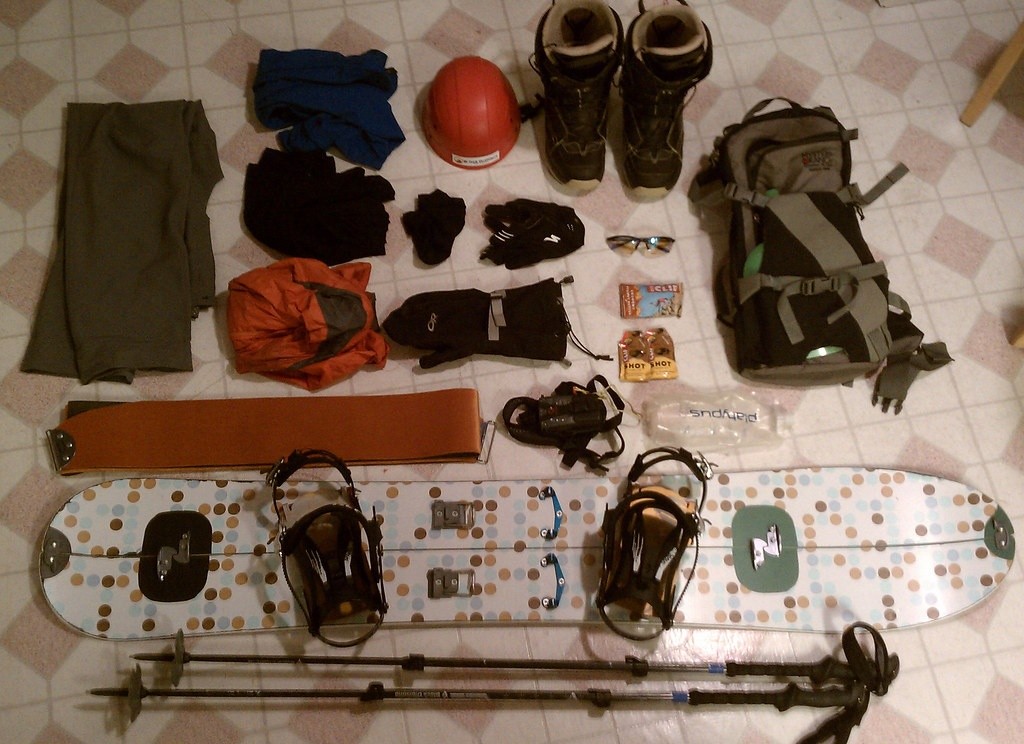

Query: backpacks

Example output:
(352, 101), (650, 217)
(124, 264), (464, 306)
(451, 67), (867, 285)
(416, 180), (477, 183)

(694, 95), (955, 416)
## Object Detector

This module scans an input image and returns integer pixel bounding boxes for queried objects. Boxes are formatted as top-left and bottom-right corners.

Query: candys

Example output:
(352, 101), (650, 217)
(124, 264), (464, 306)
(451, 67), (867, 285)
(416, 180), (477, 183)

(619, 282), (683, 318)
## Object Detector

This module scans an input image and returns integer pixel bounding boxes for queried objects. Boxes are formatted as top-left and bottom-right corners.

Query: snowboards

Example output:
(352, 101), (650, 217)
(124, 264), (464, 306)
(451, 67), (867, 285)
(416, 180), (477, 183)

(31, 461), (1019, 643)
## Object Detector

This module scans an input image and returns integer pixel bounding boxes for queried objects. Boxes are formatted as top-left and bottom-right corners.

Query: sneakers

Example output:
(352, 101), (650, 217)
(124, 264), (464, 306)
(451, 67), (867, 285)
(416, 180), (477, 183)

(613, 0), (713, 198)
(527, 0), (625, 198)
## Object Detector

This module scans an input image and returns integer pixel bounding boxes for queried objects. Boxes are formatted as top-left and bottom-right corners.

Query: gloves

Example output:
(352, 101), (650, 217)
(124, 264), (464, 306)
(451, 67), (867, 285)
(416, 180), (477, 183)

(480, 198), (585, 270)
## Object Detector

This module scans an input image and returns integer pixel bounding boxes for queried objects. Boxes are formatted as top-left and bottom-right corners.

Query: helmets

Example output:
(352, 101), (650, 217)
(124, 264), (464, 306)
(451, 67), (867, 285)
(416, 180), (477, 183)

(423, 55), (521, 170)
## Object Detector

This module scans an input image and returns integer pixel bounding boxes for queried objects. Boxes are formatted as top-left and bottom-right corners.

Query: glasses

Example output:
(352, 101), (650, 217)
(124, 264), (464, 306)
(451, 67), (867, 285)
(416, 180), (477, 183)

(606, 235), (675, 257)
